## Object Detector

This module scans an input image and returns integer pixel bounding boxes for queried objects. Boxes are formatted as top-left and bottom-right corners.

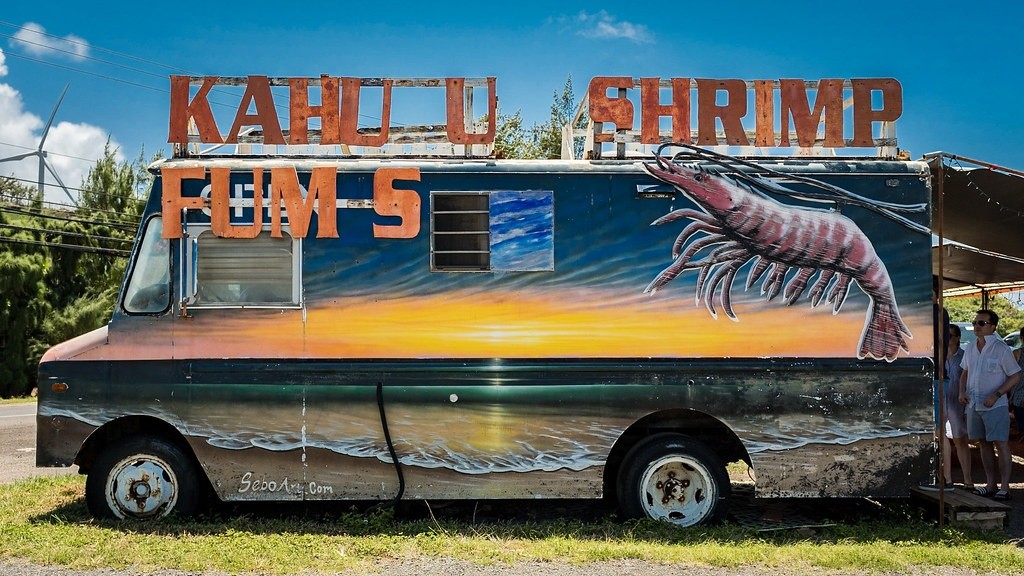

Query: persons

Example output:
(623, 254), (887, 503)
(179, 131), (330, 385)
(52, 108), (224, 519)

(918, 279), (954, 492)
(1009, 328), (1024, 443)
(945, 324), (974, 492)
(958, 310), (1022, 501)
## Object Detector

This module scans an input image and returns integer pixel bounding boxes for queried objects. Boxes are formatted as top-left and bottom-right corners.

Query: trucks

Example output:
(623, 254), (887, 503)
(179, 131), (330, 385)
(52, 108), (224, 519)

(31, 74), (938, 533)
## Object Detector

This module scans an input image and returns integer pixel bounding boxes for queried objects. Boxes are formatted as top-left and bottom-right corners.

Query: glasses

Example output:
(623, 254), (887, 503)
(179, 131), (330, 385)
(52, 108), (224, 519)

(949, 333), (958, 339)
(972, 320), (994, 326)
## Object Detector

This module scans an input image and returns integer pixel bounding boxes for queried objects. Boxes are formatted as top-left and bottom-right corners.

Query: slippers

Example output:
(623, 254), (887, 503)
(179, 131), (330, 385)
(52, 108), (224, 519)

(994, 491), (1011, 500)
(972, 486), (998, 496)
(964, 482), (974, 490)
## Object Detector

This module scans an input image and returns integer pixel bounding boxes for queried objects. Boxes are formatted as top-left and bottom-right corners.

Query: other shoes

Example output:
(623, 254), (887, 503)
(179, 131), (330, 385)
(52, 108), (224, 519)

(919, 480), (954, 491)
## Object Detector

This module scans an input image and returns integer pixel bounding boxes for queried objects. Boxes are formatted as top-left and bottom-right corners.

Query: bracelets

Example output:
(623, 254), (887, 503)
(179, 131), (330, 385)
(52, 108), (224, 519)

(994, 391), (1001, 398)
